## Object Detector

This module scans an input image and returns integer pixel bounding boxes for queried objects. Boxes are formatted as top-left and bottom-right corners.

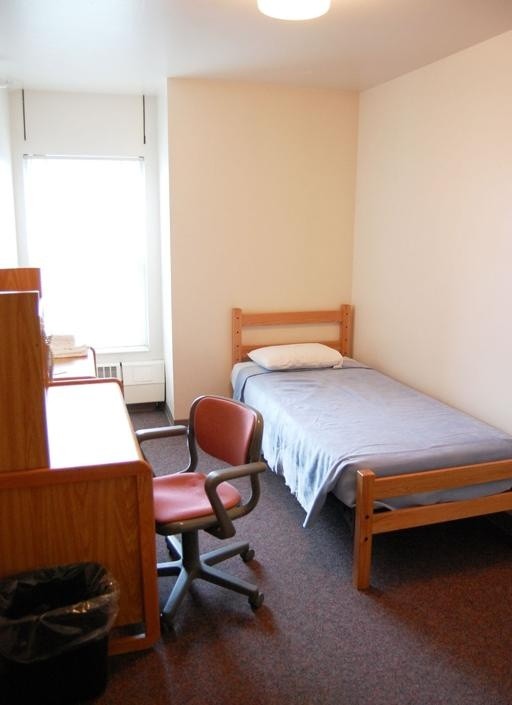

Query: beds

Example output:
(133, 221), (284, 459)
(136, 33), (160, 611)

(232, 302), (512, 591)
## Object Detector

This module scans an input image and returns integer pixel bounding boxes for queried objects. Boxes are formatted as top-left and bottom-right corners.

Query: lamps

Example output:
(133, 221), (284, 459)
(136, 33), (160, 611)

(258, 1), (332, 21)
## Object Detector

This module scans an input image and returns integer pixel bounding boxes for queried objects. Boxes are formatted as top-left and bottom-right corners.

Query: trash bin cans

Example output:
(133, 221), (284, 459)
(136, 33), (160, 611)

(0, 562), (118, 705)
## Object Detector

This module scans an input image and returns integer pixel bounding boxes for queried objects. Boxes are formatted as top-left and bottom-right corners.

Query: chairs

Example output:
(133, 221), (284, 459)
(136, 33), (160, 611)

(125, 395), (283, 629)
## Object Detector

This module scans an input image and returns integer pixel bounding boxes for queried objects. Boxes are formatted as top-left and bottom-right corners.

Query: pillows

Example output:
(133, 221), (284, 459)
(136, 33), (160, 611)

(246, 338), (339, 371)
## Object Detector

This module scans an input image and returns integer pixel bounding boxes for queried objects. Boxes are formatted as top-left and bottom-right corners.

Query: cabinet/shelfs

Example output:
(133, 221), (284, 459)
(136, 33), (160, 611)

(0, 268), (161, 659)
